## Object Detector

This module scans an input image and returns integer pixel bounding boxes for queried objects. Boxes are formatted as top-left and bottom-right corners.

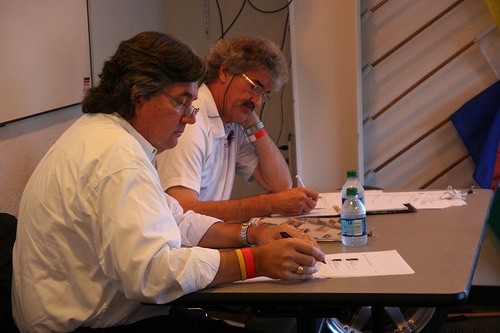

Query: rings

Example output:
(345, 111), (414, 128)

(296, 265), (304, 274)
(301, 232), (304, 236)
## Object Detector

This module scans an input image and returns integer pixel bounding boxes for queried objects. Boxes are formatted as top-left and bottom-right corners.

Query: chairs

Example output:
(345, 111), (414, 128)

(0, 213), (24, 333)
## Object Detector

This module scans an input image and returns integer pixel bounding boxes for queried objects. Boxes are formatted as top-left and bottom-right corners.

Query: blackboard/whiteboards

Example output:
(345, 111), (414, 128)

(0, 0), (94, 128)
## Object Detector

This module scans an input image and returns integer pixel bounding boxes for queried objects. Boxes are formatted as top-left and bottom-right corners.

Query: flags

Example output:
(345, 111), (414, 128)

(450, 80), (500, 240)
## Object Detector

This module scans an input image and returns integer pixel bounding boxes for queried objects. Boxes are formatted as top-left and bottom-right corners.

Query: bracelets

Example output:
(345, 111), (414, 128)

(245, 121), (264, 135)
(240, 222), (258, 248)
(235, 248), (255, 281)
(248, 129), (267, 143)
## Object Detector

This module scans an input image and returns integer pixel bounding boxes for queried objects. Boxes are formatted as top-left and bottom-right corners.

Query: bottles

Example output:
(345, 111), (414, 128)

(341, 188), (367, 247)
(341, 170), (365, 206)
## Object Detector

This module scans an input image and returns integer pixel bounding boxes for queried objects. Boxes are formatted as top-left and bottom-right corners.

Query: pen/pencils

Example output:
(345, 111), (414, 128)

(296, 175), (305, 188)
(279, 231), (326, 264)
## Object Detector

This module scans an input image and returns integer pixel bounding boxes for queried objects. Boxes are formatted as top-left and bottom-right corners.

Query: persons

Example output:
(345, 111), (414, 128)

(155, 36), (319, 221)
(11, 31), (327, 333)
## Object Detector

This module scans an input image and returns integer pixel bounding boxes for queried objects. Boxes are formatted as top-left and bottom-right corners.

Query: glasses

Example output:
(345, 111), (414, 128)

(241, 72), (271, 102)
(160, 89), (199, 117)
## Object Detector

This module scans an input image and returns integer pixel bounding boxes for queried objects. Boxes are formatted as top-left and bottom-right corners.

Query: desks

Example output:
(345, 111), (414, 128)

(150, 189), (494, 332)
